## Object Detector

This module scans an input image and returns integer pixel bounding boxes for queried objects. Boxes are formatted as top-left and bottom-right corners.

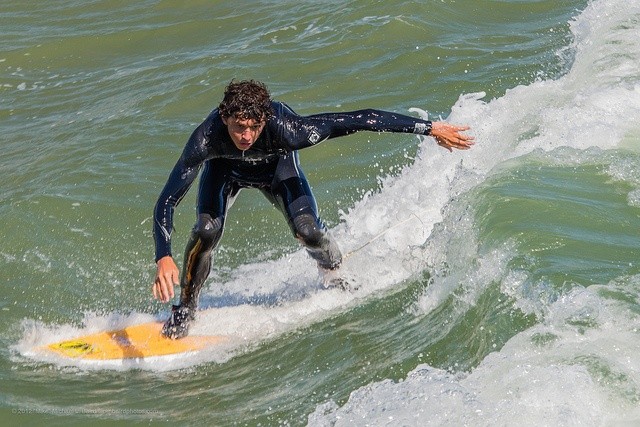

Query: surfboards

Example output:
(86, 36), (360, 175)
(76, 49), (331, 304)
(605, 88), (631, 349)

(30, 320), (231, 359)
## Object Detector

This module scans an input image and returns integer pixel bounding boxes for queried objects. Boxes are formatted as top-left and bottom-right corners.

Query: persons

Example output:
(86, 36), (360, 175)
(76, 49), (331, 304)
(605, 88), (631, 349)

(152, 81), (476, 341)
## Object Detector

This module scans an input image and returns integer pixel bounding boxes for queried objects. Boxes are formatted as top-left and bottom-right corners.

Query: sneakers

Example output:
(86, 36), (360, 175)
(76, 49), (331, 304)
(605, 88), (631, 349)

(161, 304), (200, 341)
